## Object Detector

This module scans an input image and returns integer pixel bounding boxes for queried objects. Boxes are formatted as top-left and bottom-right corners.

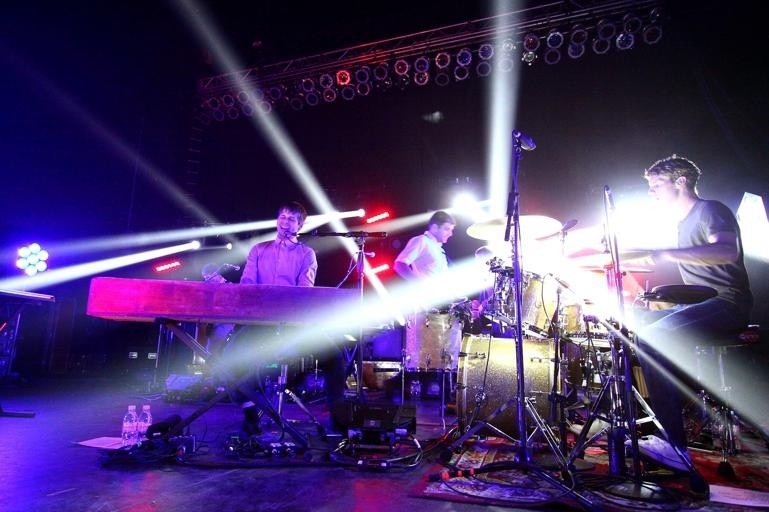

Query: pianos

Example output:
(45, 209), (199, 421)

(84, 276), (361, 329)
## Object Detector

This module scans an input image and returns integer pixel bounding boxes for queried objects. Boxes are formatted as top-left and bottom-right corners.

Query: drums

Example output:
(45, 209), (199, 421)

(456, 331), (561, 438)
(362, 360), (401, 391)
(561, 304), (623, 349)
(485, 267), (558, 338)
(404, 309), (462, 369)
(652, 284), (718, 304)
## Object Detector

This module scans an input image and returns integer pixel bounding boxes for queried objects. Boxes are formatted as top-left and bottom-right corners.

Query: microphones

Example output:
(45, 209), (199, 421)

(514, 128), (537, 151)
(549, 272), (571, 289)
(225, 263), (241, 271)
(283, 230), (298, 237)
(356, 251), (376, 257)
(605, 186), (615, 208)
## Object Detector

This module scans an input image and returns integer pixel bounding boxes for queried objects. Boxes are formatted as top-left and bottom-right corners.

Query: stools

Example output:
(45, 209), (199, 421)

(689, 325), (769, 462)
(259, 345), (326, 449)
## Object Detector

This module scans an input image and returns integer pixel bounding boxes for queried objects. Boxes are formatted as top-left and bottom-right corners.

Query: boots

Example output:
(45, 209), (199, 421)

(242, 405), (261, 436)
(331, 411), (354, 436)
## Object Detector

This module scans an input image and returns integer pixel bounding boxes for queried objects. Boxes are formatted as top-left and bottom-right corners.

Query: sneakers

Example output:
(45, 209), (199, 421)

(624, 432), (693, 472)
(571, 413), (616, 442)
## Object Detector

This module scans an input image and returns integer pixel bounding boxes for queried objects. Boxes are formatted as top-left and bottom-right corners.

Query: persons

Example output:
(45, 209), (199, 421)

(240, 200), (360, 437)
(621, 154), (757, 472)
(200, 262), (240, 365)
(382, 210), (462, 416)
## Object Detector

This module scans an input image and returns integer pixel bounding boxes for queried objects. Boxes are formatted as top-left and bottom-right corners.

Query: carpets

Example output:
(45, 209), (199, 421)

(406, 437), (769, 512)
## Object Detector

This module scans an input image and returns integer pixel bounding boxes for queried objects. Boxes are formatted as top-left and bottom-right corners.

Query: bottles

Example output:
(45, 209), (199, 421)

(120, 404), (154, 448)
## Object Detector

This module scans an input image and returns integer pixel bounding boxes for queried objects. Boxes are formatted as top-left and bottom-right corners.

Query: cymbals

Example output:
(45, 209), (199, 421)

(466, 215), (563, 240)
(592, 269), (651, 274)
(572, 251), (651, 267)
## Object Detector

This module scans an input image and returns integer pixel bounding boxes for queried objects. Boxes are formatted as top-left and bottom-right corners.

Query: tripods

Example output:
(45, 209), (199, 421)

(428, 141), (607, 512)
(561, 318), (706, 493)
(258, 362), (321, 427)
(526, 291), (589, 447)
